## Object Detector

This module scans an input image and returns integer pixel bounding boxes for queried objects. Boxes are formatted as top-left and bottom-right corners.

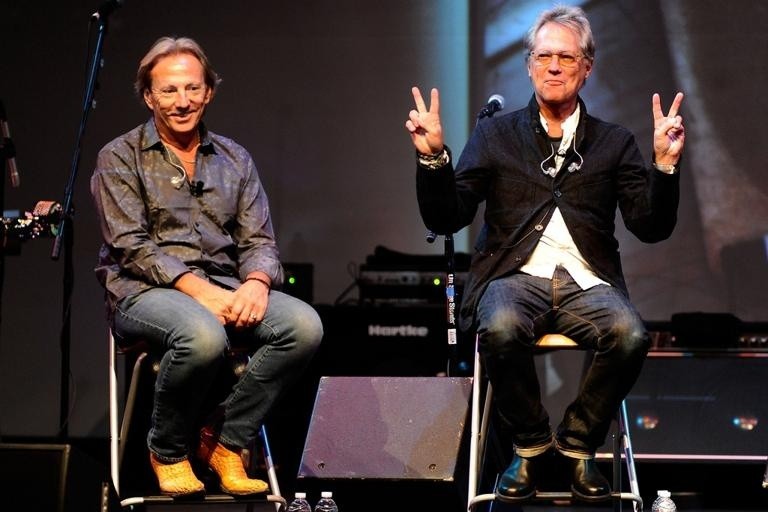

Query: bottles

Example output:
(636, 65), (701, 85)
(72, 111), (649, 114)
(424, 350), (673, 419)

(286, 492), (313, 511)
(651, 490), (678, 511)
(313, 492), (339, 511)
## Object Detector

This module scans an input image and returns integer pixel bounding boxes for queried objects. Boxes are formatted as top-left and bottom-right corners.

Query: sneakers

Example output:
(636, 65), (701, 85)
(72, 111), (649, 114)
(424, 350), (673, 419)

(148, 446), (204, 496)
(199, 426), (270, 496)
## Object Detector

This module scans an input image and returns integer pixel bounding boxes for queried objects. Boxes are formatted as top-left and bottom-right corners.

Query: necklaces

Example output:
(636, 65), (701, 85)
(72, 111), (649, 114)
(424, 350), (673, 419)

(182, 131), (200, 164)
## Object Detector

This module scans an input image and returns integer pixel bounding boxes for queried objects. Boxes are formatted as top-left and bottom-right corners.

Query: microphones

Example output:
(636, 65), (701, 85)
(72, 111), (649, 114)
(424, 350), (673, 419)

(531, 119), (540, 134)
(89, 0), (124, 22)
(0, 99), (21, 189)
(482, 94), (505, 116)
(193, 181), (203, 195)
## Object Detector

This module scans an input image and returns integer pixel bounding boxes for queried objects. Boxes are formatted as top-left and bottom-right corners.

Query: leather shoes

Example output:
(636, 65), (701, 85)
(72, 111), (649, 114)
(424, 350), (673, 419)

(496, 444), (547, 504)
(556, 447), (613, 503)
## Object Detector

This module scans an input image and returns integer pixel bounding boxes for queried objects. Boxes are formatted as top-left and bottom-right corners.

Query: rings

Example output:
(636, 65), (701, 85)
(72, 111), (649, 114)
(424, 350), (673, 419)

(250, 314), (255, 319)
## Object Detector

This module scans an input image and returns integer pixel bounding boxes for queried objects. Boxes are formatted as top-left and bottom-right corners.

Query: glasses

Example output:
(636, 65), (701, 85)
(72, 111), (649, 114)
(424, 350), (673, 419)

(529, 48), (590, 64)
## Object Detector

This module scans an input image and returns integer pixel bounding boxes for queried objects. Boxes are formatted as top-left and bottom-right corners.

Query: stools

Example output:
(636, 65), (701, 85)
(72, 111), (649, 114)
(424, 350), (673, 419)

(100, 319), (288, 512)
(467, 326), (647, 512)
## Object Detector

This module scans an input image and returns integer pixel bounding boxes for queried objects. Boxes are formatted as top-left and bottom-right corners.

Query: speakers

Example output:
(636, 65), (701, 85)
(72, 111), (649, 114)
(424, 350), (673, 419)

(296, 376), (473, 512)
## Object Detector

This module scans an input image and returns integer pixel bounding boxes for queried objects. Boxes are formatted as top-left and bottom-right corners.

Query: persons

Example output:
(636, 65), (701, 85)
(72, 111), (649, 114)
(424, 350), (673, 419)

(89, 35), (324, 495)
(406, 4), (686, 500)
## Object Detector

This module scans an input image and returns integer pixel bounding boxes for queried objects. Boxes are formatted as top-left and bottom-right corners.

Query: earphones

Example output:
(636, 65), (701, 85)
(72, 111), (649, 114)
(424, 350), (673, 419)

(548, 167), (558, 177)
(567, 162), (579, 173)
(171, 176), (183, 189)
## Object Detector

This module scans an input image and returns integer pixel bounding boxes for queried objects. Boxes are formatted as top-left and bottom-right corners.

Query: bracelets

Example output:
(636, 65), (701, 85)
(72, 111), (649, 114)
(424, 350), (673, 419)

(246, 278), (270, 294)
(417, 151), (443, 160)
